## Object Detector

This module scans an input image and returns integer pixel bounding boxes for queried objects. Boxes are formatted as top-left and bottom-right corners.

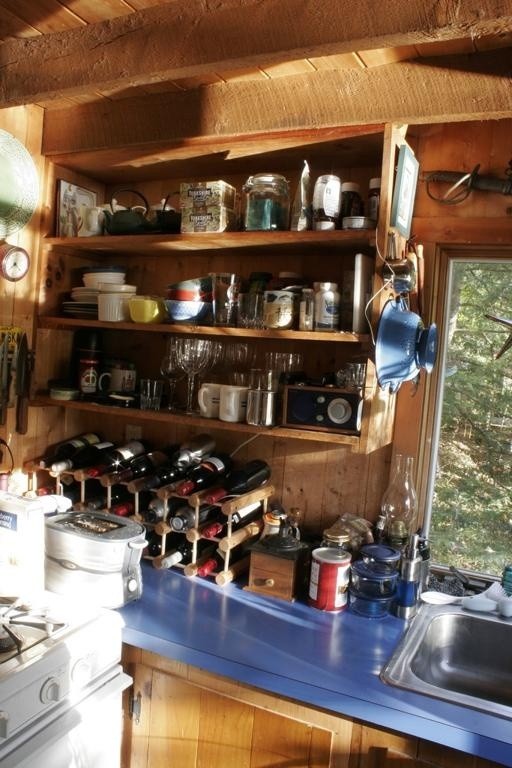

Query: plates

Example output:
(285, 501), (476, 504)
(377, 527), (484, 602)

(62, 266), (127, 318)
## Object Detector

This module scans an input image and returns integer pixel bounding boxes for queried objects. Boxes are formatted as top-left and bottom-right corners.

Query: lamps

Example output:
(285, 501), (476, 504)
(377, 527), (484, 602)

(375, 453), (423, 555)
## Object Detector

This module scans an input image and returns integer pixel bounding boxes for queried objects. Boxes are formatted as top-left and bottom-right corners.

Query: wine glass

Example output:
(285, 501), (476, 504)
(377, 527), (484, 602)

(160, 337), (254, 414)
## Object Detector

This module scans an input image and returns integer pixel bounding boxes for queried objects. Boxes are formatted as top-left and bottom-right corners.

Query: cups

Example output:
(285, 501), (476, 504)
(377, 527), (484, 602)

(237, 294), (264, 329)
(198, 382), (248, 421)
(265, 351), (305, 392)
(212, 272), (235, 325)
(140, 379), (162, 410)
(99, 369), (137, 393)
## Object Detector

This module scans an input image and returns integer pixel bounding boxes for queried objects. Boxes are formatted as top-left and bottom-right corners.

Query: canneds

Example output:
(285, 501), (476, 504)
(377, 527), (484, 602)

(306, 546), (354, 613)
(320, 527), (354, 554)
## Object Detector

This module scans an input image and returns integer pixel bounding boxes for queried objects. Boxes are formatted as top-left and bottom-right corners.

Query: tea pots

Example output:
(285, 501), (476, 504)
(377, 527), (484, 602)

(147, 198), (177, 224)
(103, 188), (152, 234)
(78, 197), (147, 237)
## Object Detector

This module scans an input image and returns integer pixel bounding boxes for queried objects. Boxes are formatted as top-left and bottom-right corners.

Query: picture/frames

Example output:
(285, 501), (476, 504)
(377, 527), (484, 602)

(53, 177), (99, 240)
(386, 142), (423, 240)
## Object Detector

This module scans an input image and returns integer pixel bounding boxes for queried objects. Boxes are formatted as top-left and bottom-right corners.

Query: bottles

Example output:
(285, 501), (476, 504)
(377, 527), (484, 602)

(143, 537), (245, 579)
(241, 173), (292, 231)
(316, 282), (339, 331)
(40, 431), (272, 503)
(73, 328), (103, 401)
(40, 475), (263, 539)
(298, 288), (315, 330)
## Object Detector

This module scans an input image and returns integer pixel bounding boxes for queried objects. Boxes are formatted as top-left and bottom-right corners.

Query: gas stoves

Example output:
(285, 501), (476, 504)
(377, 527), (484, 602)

(1, 595), (71, 666)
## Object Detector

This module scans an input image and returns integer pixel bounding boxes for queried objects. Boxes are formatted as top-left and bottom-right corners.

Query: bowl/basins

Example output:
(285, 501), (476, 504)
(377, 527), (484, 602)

(163, 275), (211, 321)
(129, 295), (165, 325)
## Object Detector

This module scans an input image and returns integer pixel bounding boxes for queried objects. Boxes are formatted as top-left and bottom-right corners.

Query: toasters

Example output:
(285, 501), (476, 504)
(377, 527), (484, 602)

(46, 511), (144, 610)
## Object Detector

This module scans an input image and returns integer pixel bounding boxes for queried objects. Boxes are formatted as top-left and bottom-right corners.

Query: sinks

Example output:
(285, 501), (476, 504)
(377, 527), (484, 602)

(379, 600), (512, 722)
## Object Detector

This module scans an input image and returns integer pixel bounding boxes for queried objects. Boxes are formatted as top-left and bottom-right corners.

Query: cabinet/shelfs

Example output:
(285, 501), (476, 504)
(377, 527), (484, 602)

(348, 723), (511, 767)
(23, 456), (275, 586)
(39, 121), (416, 460)
(122, 644), (358, 767)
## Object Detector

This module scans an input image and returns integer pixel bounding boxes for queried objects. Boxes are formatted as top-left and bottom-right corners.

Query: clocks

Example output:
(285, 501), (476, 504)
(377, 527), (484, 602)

(1, 242), (29, 282)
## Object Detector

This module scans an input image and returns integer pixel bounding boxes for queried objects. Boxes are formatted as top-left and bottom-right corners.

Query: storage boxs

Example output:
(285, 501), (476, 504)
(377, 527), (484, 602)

(175, 178), (238, 209)
(179, 206), (241, 234)
